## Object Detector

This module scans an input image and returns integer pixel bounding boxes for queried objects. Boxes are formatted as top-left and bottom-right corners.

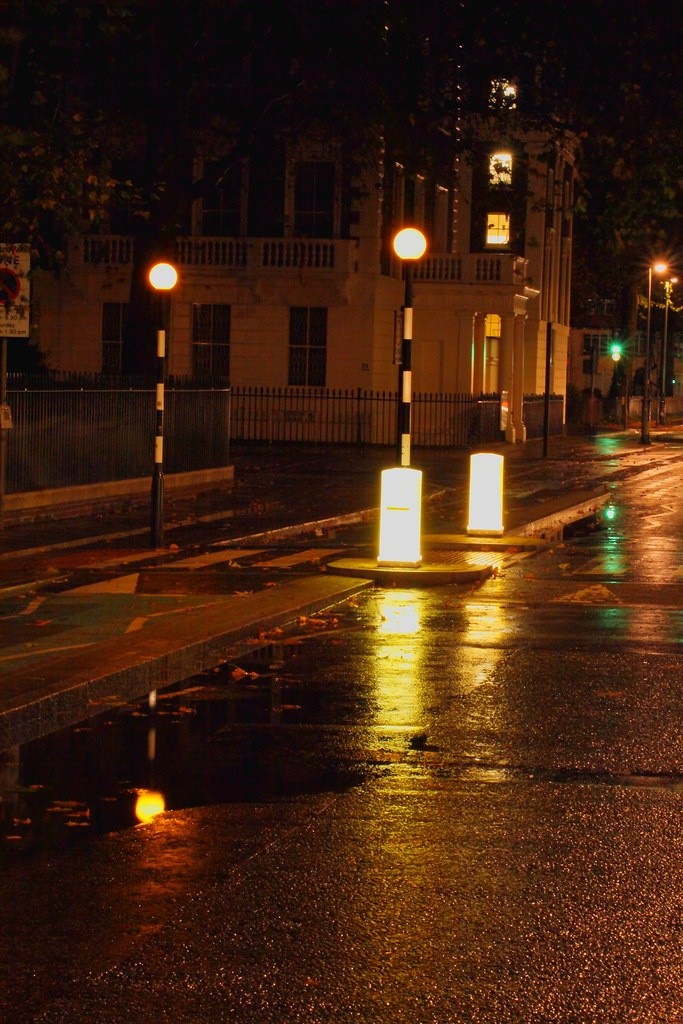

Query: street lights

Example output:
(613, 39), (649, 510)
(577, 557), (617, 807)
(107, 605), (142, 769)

(658, 272), (678, 426)
(392, 227), (427, 469)
(641, 261), (668, 447)
(145, 262), (178, 550)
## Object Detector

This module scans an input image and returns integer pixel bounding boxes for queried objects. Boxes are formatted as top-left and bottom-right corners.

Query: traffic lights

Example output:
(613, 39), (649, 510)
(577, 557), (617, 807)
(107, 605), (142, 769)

(610, 330), (623, 363)
